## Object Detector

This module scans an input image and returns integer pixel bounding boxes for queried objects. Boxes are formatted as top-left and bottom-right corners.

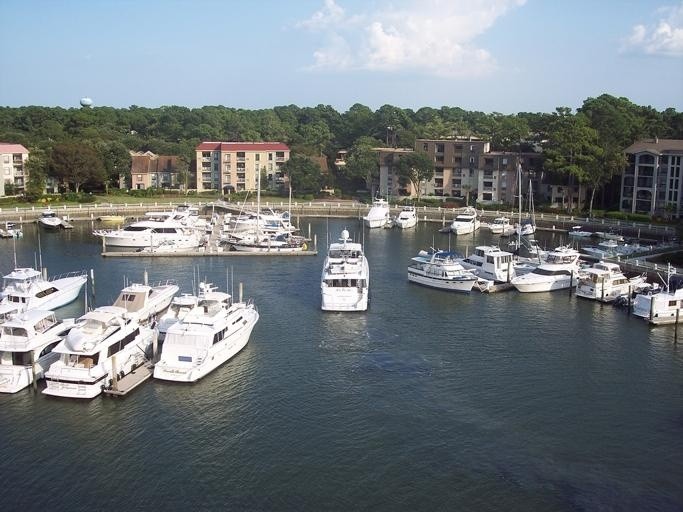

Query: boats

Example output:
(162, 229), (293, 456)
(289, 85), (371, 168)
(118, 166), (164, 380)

(359, 196), (391, 230)
(395, 205), (419, 231)
(38, 211), (62, 231)
(319, 210), (372, 314)
(92, 161), (309, 257)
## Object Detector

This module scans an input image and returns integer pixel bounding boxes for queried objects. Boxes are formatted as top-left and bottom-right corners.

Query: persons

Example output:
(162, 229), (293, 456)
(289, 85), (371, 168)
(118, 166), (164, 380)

(287, 235), (307, 251)
